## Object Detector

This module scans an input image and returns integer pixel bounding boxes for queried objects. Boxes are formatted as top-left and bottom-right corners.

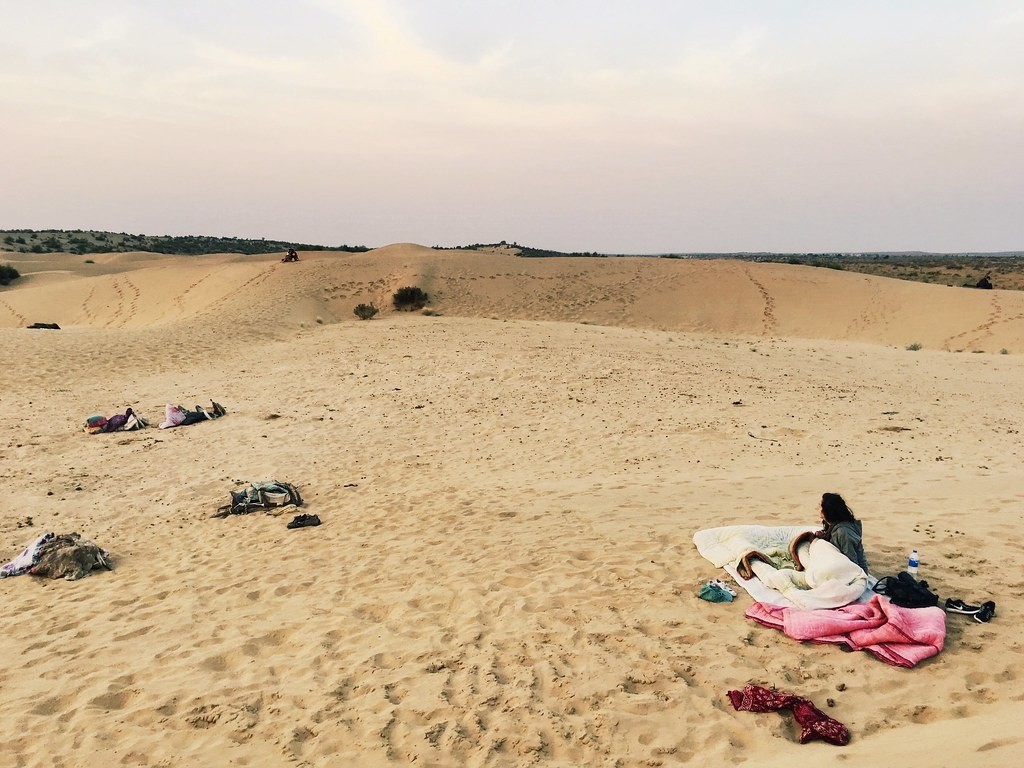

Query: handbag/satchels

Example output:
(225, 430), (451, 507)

(871, 571), (939, 608)
(699, 583), (733, 603)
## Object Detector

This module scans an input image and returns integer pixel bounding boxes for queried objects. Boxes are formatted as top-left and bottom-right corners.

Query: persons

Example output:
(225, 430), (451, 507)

(288, 247), (297, 261)
(814, 493), (868, 577)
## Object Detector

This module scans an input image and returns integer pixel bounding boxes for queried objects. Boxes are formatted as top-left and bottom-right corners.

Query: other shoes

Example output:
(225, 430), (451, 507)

(715, 579), (738, 597)
(706, 580), (716, 588)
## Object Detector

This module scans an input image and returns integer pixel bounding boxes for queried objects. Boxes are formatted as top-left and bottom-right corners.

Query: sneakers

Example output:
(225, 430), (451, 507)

(974, 600), (996, 623)
(944, 598), (981, 614)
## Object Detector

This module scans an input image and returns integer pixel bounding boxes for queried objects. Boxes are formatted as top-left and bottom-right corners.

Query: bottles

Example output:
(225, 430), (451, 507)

(908, 549), (920, 580)
